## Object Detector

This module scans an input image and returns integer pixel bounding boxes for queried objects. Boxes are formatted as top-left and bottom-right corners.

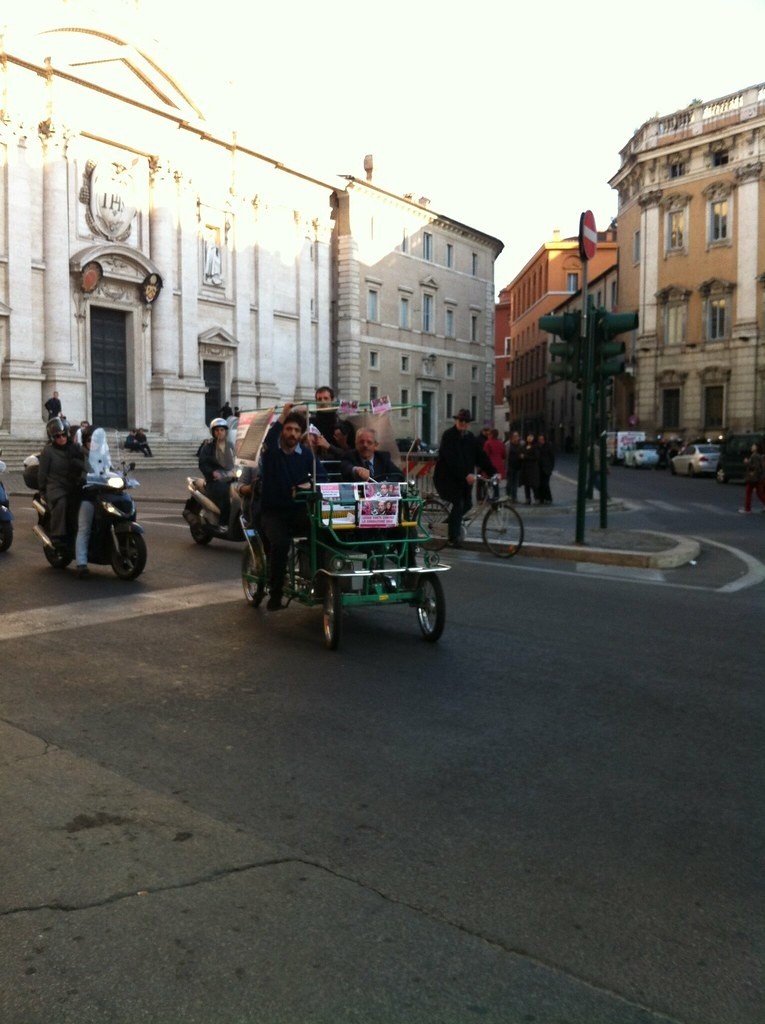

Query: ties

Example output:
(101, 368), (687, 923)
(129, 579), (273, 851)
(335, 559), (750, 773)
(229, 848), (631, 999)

(364, 461), (372, 477)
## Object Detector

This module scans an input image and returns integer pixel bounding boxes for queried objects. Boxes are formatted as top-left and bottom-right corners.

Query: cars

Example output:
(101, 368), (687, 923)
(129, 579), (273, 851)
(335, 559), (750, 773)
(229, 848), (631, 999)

(716, 433), (765, 484)
(623, 441), (660, 469)
(670, 444), (720, 478)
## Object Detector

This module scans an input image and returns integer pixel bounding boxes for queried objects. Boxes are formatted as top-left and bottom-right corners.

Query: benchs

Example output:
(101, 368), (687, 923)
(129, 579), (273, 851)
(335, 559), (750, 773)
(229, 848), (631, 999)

(317, 460), (345, 483)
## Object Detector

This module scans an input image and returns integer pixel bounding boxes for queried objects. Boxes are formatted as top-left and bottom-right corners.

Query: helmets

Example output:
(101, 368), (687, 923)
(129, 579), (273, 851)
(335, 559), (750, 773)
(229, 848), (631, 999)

(47, 417), (70, 442)
(209, 418), (229, 433)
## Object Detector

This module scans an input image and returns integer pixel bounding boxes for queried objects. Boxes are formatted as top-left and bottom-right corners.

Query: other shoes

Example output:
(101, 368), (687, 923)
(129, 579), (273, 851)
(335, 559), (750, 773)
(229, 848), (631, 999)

(446, 540), (464, 548)
(77, 565), (90, 576)
(738, 510), (751, 513)
(267, 589), (283, 612)
(507, 498), (553, 505)
(219, 525), (228, 532)
(760, 508), (765, 513)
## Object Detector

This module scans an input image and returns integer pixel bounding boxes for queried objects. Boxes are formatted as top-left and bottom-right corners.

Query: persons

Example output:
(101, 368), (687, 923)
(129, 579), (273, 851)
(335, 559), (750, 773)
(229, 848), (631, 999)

(196, 385), (418, 610)
(737, 443), (765, 513)
(476, 426), (554, 505)
(37, 392), (140, 577)
(585, 434), (610, 499)
(124, 427), (153, 457)
(432, 408), (501, 549)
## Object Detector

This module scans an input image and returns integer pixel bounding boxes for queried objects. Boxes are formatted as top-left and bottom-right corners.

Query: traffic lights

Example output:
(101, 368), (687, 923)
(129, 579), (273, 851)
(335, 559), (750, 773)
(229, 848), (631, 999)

(594, 306), (639, 379)
(538, 313), (582, 383)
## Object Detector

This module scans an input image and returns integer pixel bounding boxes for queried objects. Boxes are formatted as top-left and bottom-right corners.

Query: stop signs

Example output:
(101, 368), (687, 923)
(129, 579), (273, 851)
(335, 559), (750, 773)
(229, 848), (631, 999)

(582, 210), (597, 259)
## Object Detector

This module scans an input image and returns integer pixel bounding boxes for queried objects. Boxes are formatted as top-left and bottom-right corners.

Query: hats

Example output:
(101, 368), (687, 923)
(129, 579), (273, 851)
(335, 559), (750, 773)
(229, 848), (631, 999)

(302, 425), (321, 437)
(453, 409), (474, 422)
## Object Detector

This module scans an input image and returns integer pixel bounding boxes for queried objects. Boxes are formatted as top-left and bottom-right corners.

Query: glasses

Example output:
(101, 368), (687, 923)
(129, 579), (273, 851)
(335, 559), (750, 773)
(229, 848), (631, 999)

(55, 433), (66, 439)
(87, 438), (91, 442)
(460, 419), (469, 423)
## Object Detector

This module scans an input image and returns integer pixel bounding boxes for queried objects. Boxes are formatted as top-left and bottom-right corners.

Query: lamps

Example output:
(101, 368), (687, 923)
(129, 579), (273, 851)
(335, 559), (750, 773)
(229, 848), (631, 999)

(641, 348), (651, 352)
(739, 336), (750, 342)
(686, 343), (697, 348)
(423, 354), (436, 368)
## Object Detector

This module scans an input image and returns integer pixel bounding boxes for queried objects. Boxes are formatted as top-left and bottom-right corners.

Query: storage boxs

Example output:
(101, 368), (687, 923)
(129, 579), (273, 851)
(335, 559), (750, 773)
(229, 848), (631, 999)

(22, 452), (39, 490)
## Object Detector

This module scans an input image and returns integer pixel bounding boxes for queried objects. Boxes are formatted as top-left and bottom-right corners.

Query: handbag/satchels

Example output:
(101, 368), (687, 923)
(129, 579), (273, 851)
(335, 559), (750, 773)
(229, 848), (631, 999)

(744, 470), (758, 481)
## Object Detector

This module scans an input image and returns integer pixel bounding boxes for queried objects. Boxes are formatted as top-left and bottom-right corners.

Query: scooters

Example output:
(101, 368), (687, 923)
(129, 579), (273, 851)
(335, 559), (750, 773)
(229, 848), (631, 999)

(182, 416), (254, 545)
(23, 426), (148, 582)
(0, 449), (15, 552)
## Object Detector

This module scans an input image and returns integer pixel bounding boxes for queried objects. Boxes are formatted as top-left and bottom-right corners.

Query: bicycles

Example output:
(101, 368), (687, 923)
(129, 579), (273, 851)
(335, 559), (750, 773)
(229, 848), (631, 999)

(412, 473), (524, 558)
